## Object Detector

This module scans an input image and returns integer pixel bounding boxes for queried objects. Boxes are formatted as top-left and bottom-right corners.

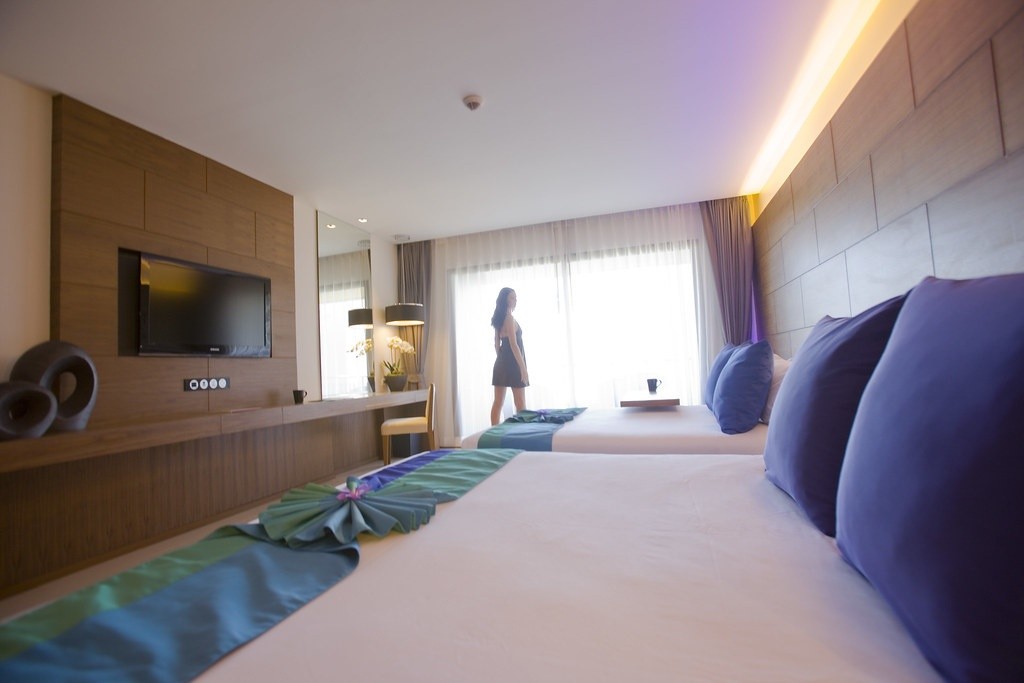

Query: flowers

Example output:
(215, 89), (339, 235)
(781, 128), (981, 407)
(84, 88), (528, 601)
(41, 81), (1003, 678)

(380, 335), (419, 376)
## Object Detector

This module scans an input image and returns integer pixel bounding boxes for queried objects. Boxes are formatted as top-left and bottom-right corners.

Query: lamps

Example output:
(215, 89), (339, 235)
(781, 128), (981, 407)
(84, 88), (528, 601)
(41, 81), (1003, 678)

(347, 309), (373, 330)
(384, 300), (426, 367)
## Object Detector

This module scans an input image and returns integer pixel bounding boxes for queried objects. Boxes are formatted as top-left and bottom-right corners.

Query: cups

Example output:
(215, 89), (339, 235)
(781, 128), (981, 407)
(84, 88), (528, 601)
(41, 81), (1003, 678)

(647, 379), (662, 392)
(292, 390), (307, 404)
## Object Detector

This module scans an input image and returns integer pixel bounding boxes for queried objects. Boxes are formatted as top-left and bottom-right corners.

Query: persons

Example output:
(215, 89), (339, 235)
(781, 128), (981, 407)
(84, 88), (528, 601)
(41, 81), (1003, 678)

(490, 287), (530, 426)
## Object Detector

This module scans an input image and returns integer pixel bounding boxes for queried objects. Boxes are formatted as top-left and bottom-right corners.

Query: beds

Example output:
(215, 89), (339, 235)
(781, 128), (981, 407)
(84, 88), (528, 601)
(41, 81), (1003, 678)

(0, 451), (948, 681)
(459, 354), (792, 454)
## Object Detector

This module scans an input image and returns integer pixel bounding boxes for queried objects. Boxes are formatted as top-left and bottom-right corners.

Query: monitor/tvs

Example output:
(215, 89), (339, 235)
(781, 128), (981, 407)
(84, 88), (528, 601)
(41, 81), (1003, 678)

(137, 254), (272, 358)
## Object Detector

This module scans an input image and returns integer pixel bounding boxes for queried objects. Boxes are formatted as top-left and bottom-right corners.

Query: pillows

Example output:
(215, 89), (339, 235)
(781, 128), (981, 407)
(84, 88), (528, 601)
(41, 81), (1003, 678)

(764, 286), (914, 538)
(713, 337), (775, 434)
(703, 339), (749, 411)
(833, 271), (1024, 683)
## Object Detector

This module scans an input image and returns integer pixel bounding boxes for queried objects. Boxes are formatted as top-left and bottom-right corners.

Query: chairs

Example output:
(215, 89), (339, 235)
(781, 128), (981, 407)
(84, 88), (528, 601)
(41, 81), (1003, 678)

(380, 384), (438, 464)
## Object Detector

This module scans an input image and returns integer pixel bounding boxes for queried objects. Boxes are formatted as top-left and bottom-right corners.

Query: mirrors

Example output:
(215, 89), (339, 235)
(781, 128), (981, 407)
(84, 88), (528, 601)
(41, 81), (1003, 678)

(316, 209), (377, 403)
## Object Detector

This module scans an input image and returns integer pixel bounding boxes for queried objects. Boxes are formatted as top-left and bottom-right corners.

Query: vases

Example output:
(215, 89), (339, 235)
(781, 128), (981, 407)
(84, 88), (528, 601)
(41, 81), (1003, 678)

(384, 375), (407, 392)
